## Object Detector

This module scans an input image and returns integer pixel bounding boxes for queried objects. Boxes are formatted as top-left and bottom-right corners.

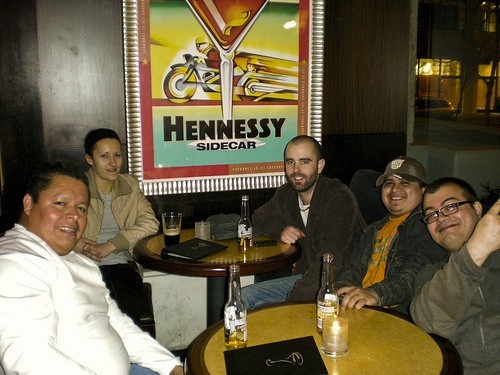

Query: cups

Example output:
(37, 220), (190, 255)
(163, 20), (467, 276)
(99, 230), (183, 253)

(321, 316), (349, 356)
(162, 212), (182, 246)
(195, 221), (210, 240)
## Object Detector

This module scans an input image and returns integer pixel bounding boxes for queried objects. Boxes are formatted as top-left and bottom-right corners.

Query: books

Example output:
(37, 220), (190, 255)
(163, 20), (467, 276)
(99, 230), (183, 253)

(161, 238), (229, 261)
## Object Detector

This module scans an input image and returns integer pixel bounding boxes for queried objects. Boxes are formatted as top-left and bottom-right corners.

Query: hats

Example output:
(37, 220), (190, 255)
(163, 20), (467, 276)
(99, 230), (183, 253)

(375, 154), (427, 186)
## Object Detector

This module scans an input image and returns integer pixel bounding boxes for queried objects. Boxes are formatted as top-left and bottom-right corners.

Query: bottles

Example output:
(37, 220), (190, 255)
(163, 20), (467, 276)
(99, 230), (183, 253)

(237, 195), (253, 251)
(223, 264), (248, 351)
(316, 253), (340, 335)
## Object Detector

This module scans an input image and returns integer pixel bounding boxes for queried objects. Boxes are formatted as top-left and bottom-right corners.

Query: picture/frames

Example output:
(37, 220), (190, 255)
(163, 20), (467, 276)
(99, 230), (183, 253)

(122, 1), (323, 196)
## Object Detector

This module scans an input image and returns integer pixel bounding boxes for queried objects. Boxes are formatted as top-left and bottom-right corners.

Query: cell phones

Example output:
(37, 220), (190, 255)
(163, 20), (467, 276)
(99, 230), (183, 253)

(254, 240), (277, 246)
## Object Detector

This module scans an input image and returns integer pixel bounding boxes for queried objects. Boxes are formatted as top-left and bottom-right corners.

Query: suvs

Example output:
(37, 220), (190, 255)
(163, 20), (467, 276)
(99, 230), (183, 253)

(414, 96), (454, 120)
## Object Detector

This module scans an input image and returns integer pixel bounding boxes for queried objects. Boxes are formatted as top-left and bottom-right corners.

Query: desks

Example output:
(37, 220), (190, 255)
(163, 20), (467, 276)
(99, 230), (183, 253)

(182, 299), (465, 375)
(131, 228), (301, 328)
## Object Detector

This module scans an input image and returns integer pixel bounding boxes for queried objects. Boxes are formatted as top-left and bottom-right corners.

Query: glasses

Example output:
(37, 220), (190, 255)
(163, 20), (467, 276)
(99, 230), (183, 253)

(423, 200), (474, 224)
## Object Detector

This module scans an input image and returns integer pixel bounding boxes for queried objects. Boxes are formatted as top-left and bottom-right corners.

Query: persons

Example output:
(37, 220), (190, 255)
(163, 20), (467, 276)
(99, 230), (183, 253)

(335, 157), (450, 316)
(409, 177), (500, 375)
(240, 134), (367, 312)
(72, 128), (161, 340)
(0, 158), (185, 375)
